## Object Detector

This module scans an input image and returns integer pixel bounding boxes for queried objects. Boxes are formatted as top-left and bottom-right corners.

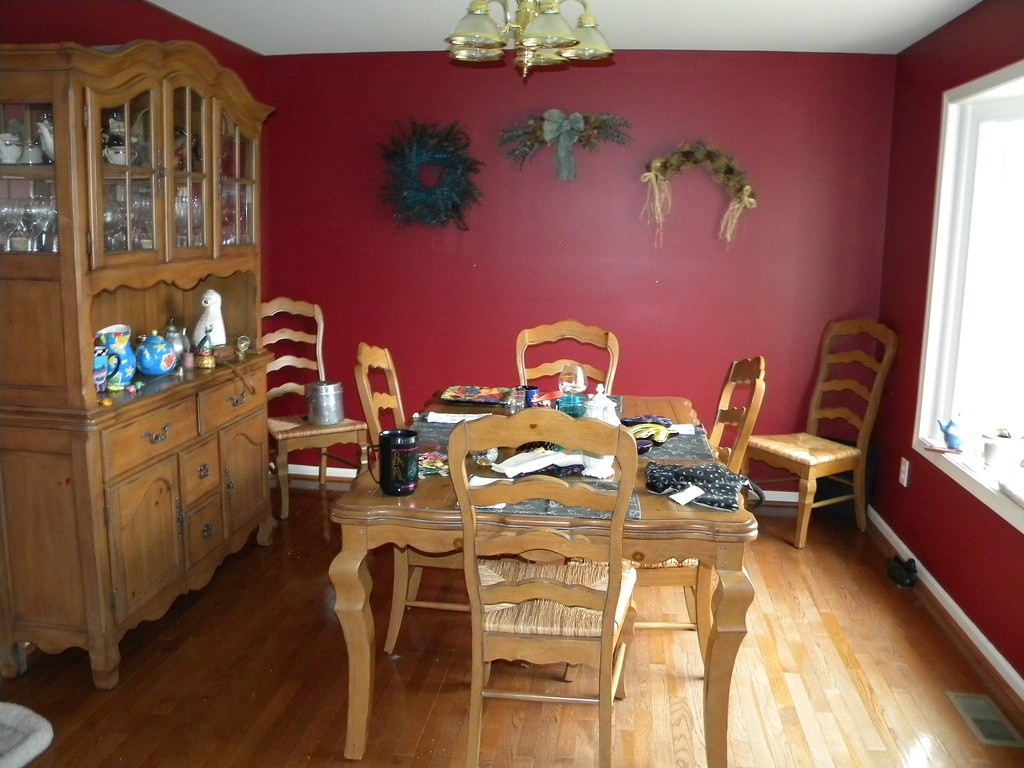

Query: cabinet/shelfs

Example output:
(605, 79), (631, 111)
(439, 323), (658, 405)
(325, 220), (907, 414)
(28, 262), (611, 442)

(0, 41), (281, 690)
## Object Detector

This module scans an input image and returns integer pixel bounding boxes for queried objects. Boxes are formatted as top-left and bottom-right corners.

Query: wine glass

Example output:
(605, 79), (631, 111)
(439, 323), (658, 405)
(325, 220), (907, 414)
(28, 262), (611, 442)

(0, 193), (246, 255)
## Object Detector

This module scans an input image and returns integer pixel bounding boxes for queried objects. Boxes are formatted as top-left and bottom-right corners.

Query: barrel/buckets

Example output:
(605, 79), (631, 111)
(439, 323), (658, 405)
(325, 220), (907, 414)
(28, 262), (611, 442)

(303, 379), (345, 426)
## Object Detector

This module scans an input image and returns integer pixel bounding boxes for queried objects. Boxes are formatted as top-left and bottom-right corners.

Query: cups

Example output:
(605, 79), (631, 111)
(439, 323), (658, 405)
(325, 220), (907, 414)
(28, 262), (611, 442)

(366, 429), (420, 497)
(559, 363), (587, 395)
(92, 346), (121, 392)
(606, 396), (623, 417)
(507, 384), (539, 416)
(0, 133), (22, 163)
(558, 395), (589, 419)
(471, 446), (499, 466)
(19, 144), (43, 164)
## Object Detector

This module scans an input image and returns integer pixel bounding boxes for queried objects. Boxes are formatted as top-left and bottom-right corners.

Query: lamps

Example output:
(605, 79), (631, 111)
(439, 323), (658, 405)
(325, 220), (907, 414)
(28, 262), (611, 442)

(445, 0), (615, 78)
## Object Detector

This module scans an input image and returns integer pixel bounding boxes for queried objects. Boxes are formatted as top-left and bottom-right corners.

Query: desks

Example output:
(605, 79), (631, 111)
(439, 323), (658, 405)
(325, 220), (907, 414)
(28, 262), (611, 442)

(329, 390), (759, 768)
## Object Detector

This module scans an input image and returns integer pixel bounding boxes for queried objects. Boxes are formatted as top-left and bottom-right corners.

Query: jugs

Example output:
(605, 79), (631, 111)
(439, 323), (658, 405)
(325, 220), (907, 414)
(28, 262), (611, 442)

(93, 323), (137, 391)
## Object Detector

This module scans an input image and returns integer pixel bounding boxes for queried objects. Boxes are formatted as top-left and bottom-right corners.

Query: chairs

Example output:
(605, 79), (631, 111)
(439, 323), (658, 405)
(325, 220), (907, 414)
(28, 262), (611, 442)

(447, 407), (637, 767)
(261, 296), (368, 519)
(353, 342), (473, 655)
(516, 320), (620, 395)
(562, 356), (765, 682)
(741, 314), (897, 548)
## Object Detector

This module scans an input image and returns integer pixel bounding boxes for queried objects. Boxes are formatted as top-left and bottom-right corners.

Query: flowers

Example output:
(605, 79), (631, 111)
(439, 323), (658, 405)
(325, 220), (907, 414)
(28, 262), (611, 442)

(645, 139), (756, 202)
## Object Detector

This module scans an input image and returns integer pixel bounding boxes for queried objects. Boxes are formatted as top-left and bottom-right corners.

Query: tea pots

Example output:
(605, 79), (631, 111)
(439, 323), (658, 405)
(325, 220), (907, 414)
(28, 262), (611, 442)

(104, 146), (138, 167)
(163, 318), (189, 367)
(136, 331), (178, 378)
(935, 414), (974, 450)
(35, 113), (54, 161)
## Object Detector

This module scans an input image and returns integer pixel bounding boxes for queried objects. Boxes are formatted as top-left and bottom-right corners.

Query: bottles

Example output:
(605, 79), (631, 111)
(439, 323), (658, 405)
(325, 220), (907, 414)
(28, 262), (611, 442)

(193, 288), (226, 355)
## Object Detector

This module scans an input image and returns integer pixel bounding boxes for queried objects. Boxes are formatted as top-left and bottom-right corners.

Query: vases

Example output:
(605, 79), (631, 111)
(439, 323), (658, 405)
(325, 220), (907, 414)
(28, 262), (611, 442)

(982, 434), (1023, 473)
